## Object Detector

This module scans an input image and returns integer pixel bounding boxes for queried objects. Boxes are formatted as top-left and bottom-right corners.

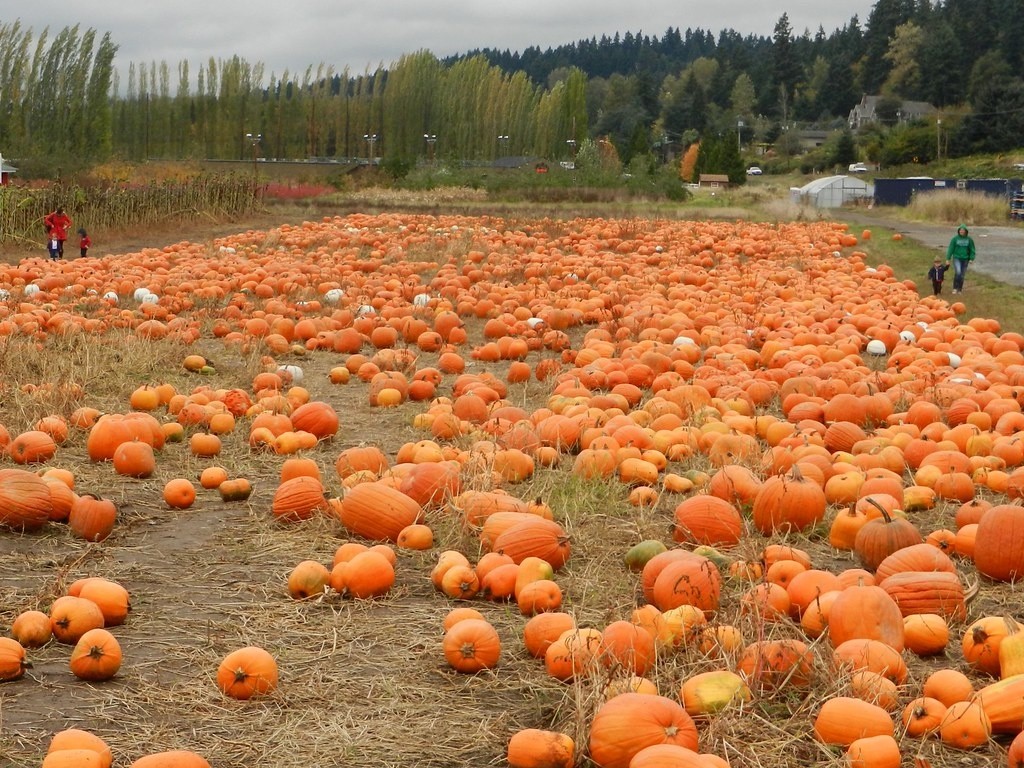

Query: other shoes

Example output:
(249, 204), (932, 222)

(952, 289), (957, 294)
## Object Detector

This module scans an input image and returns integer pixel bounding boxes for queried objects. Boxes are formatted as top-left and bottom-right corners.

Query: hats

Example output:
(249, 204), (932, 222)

(934, 255), (942, 263)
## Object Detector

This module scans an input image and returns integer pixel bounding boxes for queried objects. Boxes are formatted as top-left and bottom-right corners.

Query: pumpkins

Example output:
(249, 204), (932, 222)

(0, 213), (1024, 768)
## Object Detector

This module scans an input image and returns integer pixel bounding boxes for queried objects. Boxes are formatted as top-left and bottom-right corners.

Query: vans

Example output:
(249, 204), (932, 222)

(560, 162), (574, 170)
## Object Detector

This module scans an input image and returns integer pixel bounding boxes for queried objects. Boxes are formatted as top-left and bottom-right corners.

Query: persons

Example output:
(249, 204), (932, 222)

(47, 233), (62, 258)
(928, 255), (950, 296)
(78, 229), (91, 258)
(945, 224), (975, 296)
(44, 208), (72, 259)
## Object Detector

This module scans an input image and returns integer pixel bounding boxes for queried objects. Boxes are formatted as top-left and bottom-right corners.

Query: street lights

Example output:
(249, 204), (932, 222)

(567, 139), (575, 160)
(498, 135), (508, 156)
(424, 134), (436, 165)
(364, 134), (376, 169)
(247, 134), (261, 187)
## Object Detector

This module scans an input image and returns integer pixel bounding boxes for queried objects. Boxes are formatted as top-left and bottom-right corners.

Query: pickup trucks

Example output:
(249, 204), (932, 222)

(747, 167), (762, 175)
(849, 164), (866, 172)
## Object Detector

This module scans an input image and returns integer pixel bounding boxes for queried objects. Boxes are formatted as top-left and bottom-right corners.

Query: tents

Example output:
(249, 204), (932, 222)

(798, 174), (876, 210)
